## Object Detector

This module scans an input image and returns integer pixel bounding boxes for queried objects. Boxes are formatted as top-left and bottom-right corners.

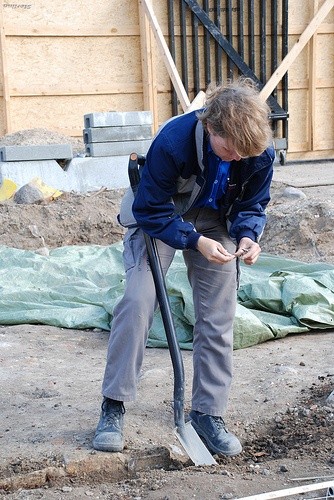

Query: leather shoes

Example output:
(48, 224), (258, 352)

(190, 409), (243, 457)
(90, 401), (126, 452)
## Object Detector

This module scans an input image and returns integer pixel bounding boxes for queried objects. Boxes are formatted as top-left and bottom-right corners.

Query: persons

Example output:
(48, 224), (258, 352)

(92, 78), (275, 456)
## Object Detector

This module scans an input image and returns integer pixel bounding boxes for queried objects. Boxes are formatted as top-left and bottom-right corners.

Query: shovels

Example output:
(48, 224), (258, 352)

(129, 154), (218, 468)
